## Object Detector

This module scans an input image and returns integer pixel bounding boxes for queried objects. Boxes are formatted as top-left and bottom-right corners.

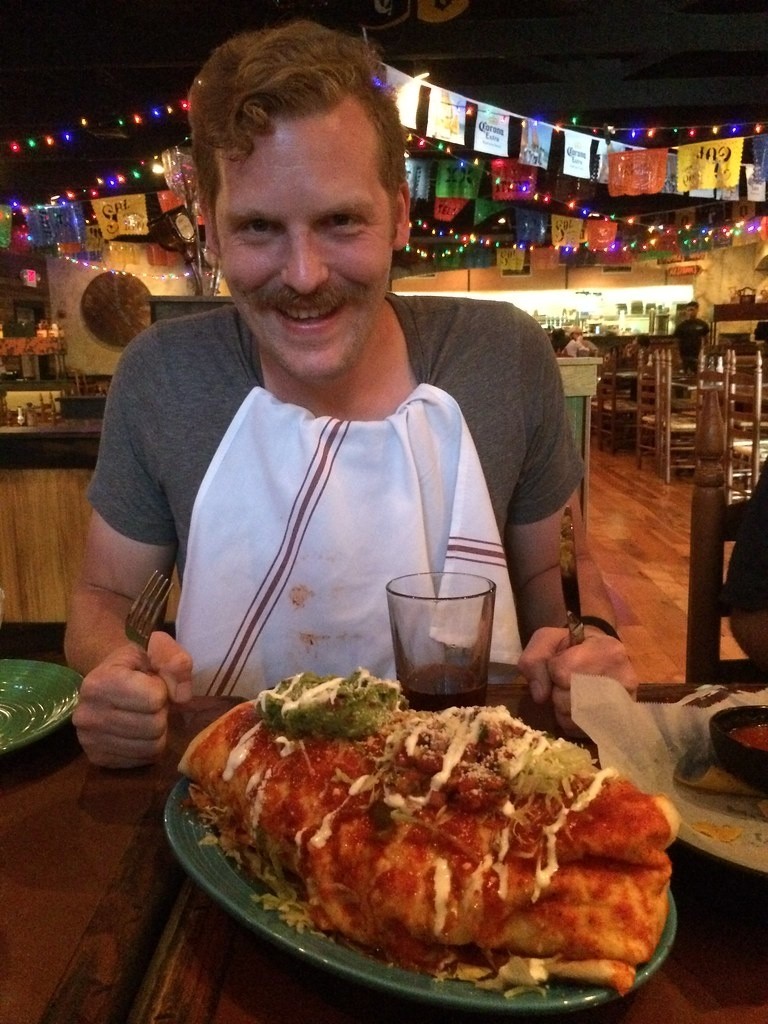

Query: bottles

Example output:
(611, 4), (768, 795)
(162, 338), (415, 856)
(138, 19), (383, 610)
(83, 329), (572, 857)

(27, 403), (36, 425)
(17, 406), (25, 426)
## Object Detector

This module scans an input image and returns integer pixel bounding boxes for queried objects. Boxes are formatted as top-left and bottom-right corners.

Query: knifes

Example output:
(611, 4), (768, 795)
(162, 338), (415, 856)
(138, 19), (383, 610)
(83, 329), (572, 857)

(560, 505), (584, 646)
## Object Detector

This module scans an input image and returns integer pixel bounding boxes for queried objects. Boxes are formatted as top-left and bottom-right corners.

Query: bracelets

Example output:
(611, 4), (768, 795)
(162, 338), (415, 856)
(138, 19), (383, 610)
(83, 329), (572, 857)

(564, 615), (623, 644)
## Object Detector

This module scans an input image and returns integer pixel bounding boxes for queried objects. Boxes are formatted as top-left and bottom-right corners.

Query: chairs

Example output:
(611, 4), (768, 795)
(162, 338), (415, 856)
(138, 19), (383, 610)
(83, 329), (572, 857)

(557, 339), (768, 685)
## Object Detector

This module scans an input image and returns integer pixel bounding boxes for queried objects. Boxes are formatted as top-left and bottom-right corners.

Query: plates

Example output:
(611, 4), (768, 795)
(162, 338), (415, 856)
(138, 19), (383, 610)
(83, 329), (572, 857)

(0, 659), (84, 754)
(165, 775), (676, 1008)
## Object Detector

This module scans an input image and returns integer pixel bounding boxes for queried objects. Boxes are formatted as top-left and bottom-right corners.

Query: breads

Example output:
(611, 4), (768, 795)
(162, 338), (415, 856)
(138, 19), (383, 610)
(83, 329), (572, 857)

(184, 692), (675, 989)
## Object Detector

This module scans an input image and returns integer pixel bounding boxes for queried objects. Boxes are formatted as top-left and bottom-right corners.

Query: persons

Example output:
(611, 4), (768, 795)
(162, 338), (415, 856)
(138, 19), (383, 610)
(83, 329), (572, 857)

(552, 326), (650, 371)
(725, 458), (768, 673)
(64, 22), (639, 769)
(671, 302), (709, 374)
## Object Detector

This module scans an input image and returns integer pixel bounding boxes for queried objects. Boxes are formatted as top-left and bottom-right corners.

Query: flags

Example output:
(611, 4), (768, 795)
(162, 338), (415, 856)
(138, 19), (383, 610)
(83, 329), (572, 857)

(0, 62), (768, 278)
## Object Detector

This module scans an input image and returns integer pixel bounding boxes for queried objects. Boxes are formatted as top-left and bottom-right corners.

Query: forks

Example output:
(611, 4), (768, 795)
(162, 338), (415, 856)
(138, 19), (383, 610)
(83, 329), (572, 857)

(126, 570), (174, 674)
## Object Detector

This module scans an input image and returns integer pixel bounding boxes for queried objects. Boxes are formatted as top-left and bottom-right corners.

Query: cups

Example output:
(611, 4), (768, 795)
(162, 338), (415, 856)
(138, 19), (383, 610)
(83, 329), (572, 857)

(388, 572), (497, 711)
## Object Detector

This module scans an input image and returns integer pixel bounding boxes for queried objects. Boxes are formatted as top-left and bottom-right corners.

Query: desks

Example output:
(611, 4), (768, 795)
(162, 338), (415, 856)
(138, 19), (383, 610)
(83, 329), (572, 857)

(0, 695), (768, 1024)
(606, 370), (696, 379)
(672, 380), (767, 392)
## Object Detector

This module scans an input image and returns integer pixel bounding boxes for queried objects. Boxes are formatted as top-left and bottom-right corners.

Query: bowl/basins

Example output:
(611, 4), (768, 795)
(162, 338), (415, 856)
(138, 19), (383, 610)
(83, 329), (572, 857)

(710, 705), (768, 797)
